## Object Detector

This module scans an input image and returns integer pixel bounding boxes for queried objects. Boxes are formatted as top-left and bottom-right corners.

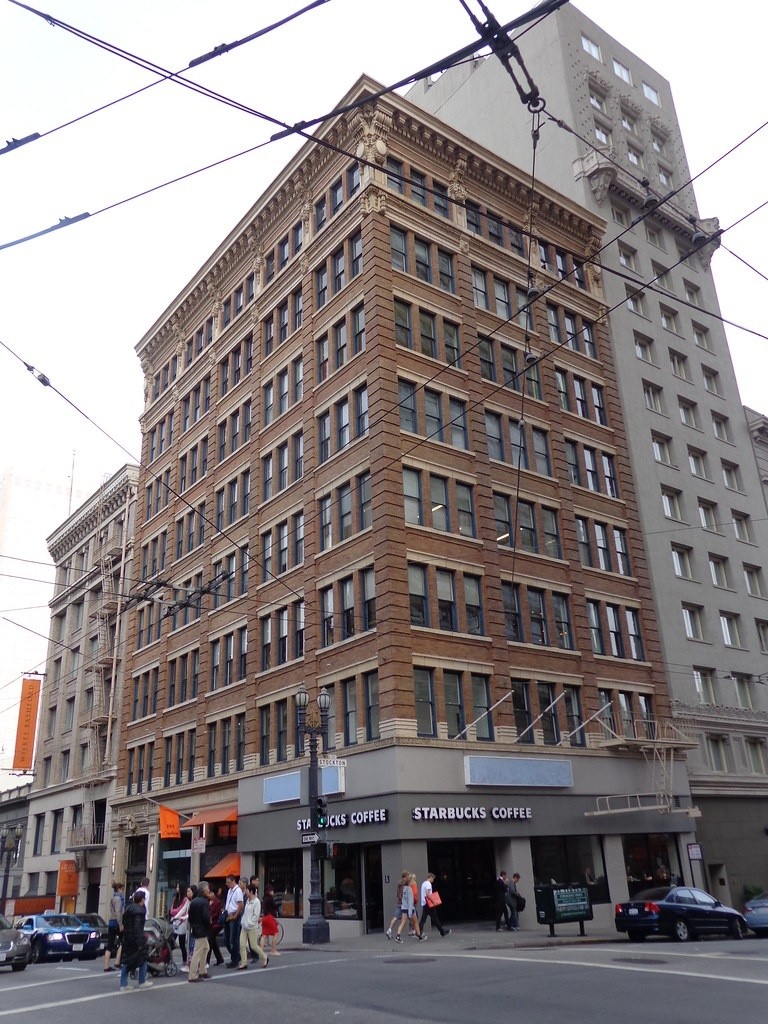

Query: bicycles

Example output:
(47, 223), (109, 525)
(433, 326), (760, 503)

(246, 911), (285, 956)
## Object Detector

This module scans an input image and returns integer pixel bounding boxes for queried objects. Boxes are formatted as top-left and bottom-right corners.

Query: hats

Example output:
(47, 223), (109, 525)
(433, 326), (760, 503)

(265, 884), (273, 893)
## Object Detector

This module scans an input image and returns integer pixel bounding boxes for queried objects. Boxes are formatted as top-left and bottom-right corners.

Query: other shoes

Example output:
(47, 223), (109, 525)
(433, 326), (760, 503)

(214, 961), (224, 966)
(115, 964), (122, 969)
(497, 925), (520, 932)
(236, 965), (247, 969)
(179, 965), (190, 972)
(199, 974), (211, 978)
(386, 930), (393, 940)
(263, 958), (269, 967)
(227, 962), (236, 968)
(189, 979), (204, 982)
(441, 929), (451, 937)
(271, 952), (281, 956)
(396, 937), (404, 944)
(139, 980), (153, 987)
(250, 958), (259, 964)
(104, 967), (115, 972)
(120, 985), (135, 992)
(408, 930), (427, 942)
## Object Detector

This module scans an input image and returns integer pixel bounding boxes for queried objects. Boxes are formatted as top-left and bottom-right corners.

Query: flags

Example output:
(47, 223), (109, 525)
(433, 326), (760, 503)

(159, 804), (181, 839)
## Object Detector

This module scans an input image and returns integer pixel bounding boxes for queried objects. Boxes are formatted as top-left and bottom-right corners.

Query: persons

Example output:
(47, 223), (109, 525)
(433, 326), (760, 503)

(626, 864), (631, 881)
(129, 878), (151, 920)
(581, 867), (595, 883)
(506, 873), (521, 932)
(171, 874), (281, 982)
(494, 870), (511, 932)
(415, 873), (451, 940)
(104, 883), (125, 972)
(385, 871), (427, 944)
(119, 891), (154, 991)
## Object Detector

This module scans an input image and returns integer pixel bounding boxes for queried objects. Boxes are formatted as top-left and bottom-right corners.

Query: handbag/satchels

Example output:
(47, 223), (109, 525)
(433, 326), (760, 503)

(218, 910), (227, 923)
(426, 892), (442, 908)
(517, 897), (526, 911)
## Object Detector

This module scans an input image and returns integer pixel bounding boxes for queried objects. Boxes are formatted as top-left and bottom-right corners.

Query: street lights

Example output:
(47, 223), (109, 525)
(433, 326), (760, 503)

(0, 821), (24, 917)
(295, 682), (332, 946)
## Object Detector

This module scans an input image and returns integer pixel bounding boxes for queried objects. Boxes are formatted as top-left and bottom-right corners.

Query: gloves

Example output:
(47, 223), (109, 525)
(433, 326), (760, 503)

(138, 945), (143, 952)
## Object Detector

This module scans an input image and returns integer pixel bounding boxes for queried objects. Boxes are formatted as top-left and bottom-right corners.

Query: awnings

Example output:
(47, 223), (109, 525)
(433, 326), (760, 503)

(204, 853), (240, 877)
(182, 805), (237, 826)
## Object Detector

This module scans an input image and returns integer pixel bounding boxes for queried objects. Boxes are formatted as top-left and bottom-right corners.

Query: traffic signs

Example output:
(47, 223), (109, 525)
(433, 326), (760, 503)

(302, 832), (321, 844)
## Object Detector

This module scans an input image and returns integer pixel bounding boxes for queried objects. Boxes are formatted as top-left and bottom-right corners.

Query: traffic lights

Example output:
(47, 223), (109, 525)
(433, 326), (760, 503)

(317, 796), (329, 826)
(314, 843), (330, 860)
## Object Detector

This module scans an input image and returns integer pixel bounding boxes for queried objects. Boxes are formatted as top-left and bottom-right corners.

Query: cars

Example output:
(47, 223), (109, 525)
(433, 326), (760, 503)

(13, 909), (101, 965)
(615, 885), (749, 942)
(741, 891), (768, 939)
(61, 911), (121, 958)
(0, 912), (32, 972)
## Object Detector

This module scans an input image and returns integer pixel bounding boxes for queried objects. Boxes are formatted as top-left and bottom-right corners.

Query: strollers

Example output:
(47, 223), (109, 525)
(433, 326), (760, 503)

(129, 917), (184, 981)
